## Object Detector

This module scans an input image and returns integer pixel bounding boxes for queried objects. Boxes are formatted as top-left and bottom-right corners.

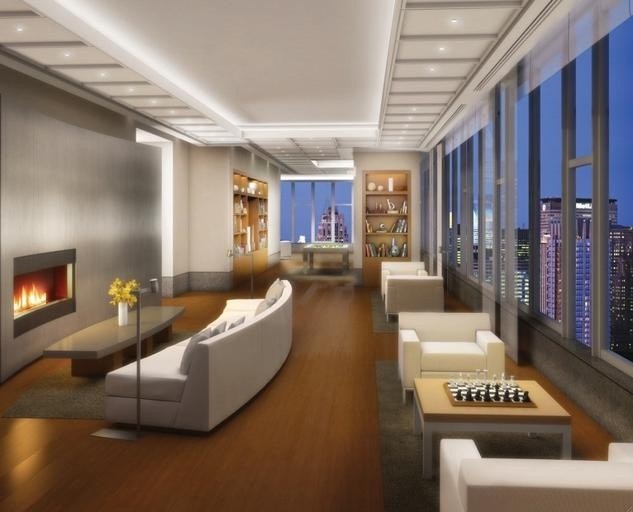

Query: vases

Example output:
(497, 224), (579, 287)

(107, 278), (141, 308)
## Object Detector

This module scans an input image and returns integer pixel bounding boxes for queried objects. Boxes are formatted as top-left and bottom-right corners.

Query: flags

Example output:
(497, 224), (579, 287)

(118, 302), (128, 326)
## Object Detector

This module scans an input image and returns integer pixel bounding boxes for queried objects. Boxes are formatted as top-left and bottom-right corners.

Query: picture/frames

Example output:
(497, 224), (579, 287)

(414, 378), (572, 477)
(302, 242), (349, 275)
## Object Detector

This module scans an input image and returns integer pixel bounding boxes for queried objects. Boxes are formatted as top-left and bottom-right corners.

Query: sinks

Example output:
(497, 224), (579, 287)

(105, 278), (295, 435)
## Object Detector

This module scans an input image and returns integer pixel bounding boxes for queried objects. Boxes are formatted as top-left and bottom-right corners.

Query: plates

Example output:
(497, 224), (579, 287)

(362, 170), (412, 289)
(233, 169), (268, 286)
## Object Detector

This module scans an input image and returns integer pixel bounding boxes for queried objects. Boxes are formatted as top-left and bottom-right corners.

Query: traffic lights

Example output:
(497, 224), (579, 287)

(180, 279), (286, 376)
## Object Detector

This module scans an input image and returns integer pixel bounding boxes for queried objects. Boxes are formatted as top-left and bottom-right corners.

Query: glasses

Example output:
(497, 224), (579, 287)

(91, 279), (159, 441)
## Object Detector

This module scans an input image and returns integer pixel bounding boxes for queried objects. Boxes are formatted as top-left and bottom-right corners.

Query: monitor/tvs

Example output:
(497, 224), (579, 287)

(391, 219), (406, 232)
(365, 243), (391, 257)
(234, 199), (268, 253)
(402, 242), (408, 256)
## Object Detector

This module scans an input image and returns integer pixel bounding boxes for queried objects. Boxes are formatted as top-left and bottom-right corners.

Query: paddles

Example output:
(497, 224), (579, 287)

(376, 359), (584, 511)
(0, 327), (198, 420)
(372, 282), (446, 333)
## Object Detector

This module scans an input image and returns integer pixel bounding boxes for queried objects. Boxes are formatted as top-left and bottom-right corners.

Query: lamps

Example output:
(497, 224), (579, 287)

(398, 310), (505, 406)
(381, 261), (445, 321)
(440, 439), (633, 512)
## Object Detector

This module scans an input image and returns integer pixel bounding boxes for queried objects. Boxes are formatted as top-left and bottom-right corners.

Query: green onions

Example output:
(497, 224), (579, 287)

(444, 367), (538, 409)
